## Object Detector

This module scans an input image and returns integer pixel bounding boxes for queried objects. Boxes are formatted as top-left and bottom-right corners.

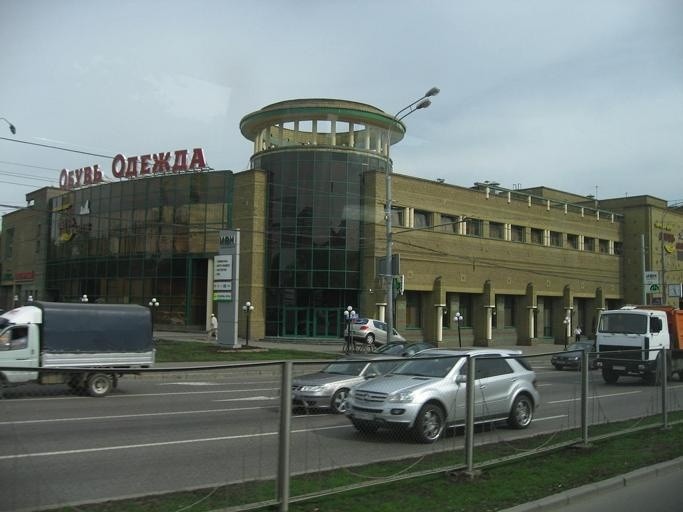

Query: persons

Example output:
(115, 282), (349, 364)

(209, 314), (219, 340)
(574, 326), (583, 342)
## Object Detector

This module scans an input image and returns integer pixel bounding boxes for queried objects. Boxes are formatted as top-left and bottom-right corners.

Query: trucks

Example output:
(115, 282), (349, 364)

(594, 304), (682, 385)
(0, 301), (156, 395)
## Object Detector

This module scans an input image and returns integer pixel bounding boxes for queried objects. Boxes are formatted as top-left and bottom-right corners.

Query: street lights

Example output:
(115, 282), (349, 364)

(562, 317), (570, 350)
(80, 294), (87, 304)
(452, 312), (463, 348)
(26, 295), (33, 302)
(343, 305), (355, 353)
(12, 293), (18, 308)
(0, 117), (16, 135)
(383, 85), (442, 339)
(241, 300), (254, 348)
(148, 298), (158, 337)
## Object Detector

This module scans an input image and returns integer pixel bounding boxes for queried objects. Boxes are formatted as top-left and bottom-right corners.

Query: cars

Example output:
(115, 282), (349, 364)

(158, 311), (190, 327)
(550, 342), (596, 371)
(288, 354), (406, 413)
(347, 341), (435, 375)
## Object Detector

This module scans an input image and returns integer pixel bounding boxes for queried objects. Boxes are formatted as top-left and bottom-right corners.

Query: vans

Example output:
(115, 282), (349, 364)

(343, 318), (405, 345)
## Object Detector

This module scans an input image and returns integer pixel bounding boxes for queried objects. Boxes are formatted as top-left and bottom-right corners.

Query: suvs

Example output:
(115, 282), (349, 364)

(345, 345), (541, 442)
